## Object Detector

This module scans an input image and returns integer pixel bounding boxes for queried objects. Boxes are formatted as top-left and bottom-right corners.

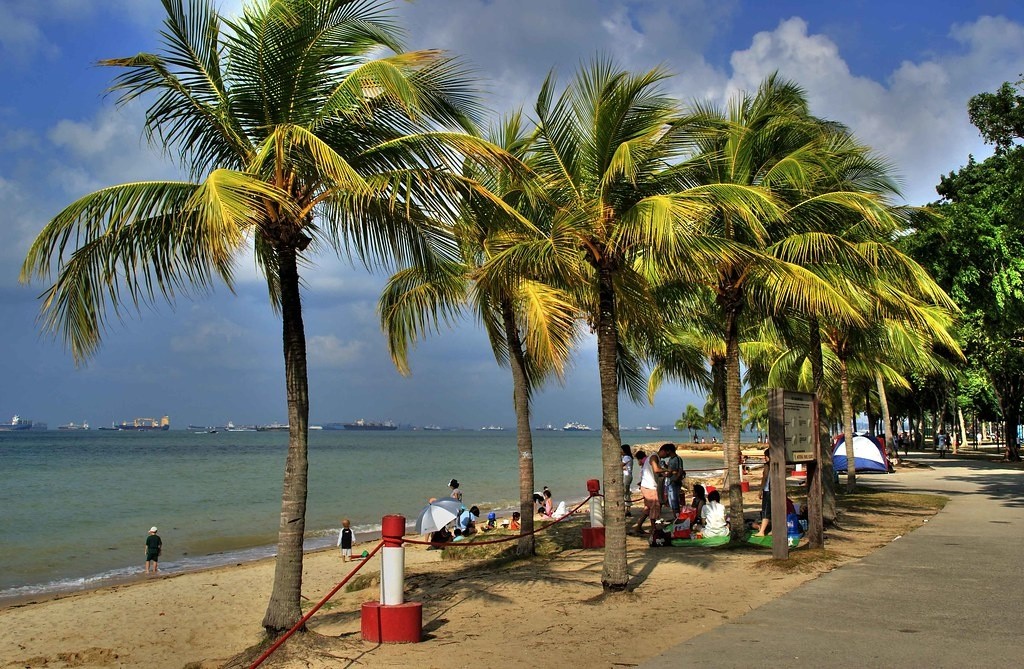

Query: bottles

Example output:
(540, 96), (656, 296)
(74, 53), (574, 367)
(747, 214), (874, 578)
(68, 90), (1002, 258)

(802, 520), (806, 531)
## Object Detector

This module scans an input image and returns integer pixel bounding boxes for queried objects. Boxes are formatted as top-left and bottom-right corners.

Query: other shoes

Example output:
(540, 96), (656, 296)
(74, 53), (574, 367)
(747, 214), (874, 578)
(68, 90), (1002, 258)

(625, 510), (631, 517)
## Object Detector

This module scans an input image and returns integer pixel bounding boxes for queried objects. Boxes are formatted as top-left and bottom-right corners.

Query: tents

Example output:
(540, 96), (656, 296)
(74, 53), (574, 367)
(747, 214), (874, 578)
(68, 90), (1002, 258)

(830, 434), (894, 474)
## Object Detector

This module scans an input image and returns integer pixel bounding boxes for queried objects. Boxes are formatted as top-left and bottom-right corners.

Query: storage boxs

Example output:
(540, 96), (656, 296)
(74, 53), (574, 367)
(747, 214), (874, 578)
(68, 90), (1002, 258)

(668, 519), (690, 538)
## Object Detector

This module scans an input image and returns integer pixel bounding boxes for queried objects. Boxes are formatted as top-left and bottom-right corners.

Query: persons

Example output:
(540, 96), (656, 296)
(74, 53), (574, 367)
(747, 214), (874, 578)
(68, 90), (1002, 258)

(937, 429), (948, 458)
(634, 443), (731, 548)
(903, 431), (909, 456)
(893, 430), (900, 456)
(620, 443), (634, 518)
(753, 448), (772, 537)
(700, 436), (705, 443)
(711, 435), (716, 444)
(693, 434), (700, 443)
(422, 478), (481, 551)
(144, 525), (162, 575)
(976, 431), (983, 445)
(510, 510), (521, 532)
(481, 511), (496, 532)
(336, 519), (356, 563)
(533, 486), (554, 521)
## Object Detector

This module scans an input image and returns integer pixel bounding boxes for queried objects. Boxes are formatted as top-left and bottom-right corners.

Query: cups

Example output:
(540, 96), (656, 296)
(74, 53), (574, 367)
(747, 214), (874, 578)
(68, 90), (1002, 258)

(697, 535), (701, 539)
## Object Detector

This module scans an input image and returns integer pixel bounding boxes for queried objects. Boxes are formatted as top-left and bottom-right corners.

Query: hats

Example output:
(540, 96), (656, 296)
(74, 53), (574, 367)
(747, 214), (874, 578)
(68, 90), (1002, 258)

(149, 526), (158, 532)
(488, 512), (497, 521)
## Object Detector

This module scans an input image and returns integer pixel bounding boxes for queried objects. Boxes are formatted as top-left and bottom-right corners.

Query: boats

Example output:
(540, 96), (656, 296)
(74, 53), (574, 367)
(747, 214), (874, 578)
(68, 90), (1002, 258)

(57, 420), (90, 429)
(535, 425), (553, 430)
(423, 424), (441, 430)
(564, 422), (591, 431)
(322, 419), (398, 430)
(99, 428), (119, 430)
(120, 416), (170, 431)
(645, 424), (661, 430)
(0, 414), (32, 431)
(482, 425), (504, 431)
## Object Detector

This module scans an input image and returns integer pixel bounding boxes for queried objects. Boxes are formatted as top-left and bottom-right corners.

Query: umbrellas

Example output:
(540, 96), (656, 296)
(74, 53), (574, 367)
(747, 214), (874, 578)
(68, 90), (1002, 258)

(414, 497), (462, 536)
